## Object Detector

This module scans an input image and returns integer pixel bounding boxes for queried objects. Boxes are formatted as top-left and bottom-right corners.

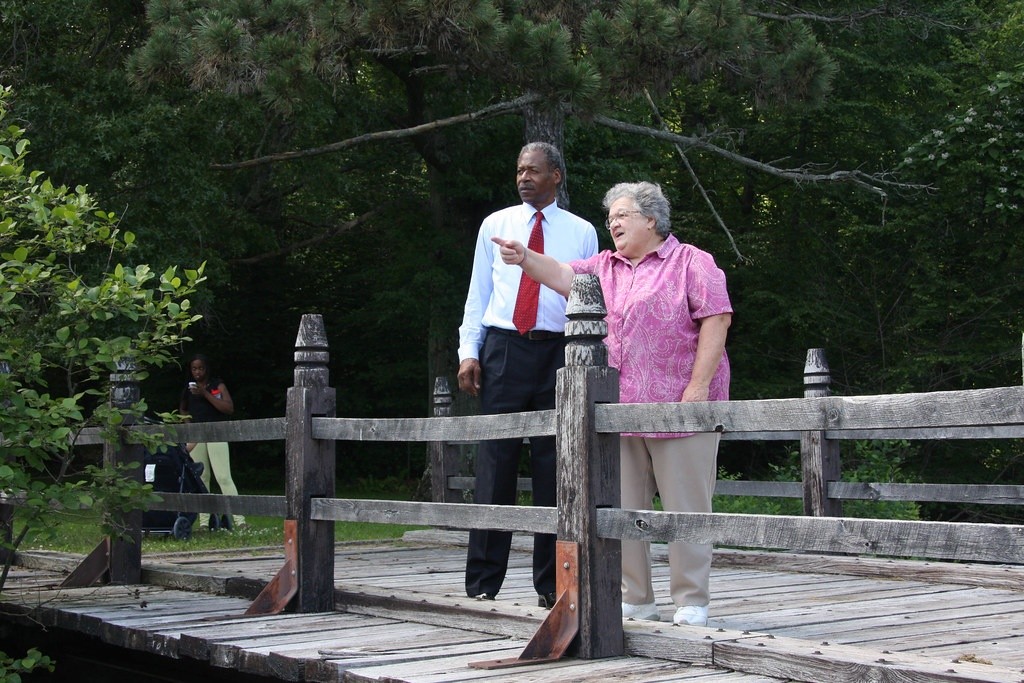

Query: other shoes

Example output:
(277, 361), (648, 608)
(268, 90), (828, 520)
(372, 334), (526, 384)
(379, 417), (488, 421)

(200, 525), (209, 530)
(239, 524), (249, 530)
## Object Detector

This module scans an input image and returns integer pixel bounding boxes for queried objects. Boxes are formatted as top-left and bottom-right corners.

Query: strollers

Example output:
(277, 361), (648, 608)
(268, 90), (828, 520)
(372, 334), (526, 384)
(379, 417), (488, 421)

(141, 416), (232, 542)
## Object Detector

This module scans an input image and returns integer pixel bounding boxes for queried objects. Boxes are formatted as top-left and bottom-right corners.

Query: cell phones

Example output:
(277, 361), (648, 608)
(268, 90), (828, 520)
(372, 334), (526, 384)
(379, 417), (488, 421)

(189, 382), (197, 394)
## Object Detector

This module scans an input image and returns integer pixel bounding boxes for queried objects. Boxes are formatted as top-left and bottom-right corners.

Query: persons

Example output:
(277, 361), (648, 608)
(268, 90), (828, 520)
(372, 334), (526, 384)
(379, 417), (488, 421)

(182, 354), (247, 530)
(490, 182), (732, 627)
(458, 142), (598, 609)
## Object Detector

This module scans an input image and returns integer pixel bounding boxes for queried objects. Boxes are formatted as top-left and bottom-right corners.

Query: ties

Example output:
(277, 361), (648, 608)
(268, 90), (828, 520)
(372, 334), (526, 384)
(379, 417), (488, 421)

(512, 212), (544, 335)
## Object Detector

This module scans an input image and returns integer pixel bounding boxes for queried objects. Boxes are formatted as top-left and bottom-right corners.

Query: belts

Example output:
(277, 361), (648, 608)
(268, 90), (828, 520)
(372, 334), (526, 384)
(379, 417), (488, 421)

(490, 327), (565, 340)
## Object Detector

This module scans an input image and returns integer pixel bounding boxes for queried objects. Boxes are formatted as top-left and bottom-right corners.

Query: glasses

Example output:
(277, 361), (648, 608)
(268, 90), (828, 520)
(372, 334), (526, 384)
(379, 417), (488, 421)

(605, 211), (641, 231)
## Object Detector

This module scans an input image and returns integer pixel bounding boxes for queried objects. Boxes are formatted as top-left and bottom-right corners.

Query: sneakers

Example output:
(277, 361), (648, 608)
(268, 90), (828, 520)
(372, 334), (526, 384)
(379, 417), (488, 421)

(538, 593), (556, 608)
(622, 602), (660, 621)
(475, 593), (495, 600)
(673, 604), (708, 627)
